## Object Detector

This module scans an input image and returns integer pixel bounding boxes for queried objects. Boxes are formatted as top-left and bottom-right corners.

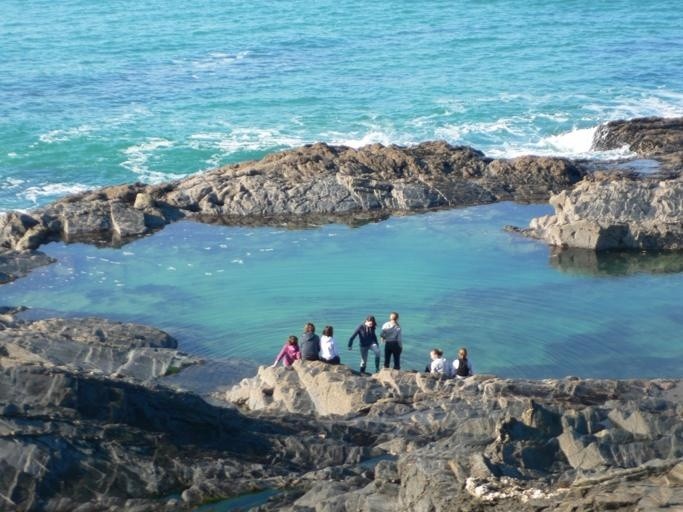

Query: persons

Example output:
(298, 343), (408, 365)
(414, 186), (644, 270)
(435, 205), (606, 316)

(299, 323), (319, 361)
(348, 316), (380, 376)
(381, 312), (402, 369)
(271, 335), (301, 369)
(318, 325), (340, 365)
(449, 348), (473, 376)
(424, 348), (450, 376)
